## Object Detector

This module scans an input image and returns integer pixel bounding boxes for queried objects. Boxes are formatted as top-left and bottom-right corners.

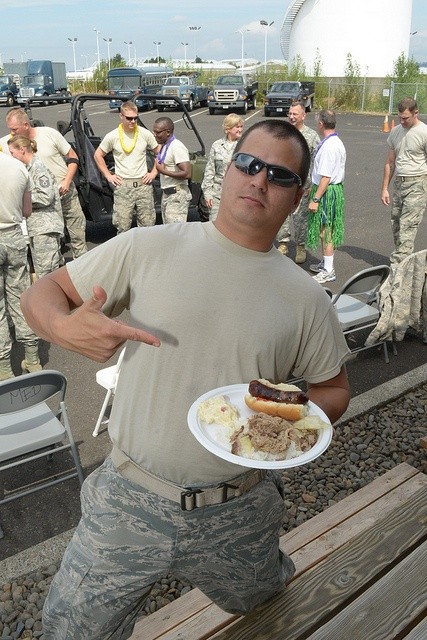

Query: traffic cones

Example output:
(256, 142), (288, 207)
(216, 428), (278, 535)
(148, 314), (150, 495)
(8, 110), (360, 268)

(383, 115), (390, 132)
(391, 120), (395, 130)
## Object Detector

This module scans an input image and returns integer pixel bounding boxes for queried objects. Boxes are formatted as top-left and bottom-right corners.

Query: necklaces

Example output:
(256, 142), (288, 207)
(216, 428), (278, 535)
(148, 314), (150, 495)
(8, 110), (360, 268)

(312, 132), (338, 158)
(119, 121), (139, 154)
(158, 134), (175, 163)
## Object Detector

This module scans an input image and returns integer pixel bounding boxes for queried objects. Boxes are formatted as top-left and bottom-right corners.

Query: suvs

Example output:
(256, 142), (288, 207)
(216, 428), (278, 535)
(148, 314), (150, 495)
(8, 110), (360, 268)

(24, 94), (209, 244)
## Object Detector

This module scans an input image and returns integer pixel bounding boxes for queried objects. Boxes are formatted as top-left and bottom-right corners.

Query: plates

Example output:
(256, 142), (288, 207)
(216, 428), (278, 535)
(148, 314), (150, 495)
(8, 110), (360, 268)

(186, 383), (334, 470)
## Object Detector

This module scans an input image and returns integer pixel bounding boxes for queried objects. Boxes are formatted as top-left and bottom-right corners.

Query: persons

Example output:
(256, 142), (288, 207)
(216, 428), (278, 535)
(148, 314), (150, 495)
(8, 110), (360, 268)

(381, 99), (427, 273)
(4, 108), (86, 268)
(21, 121), (350, 639)
(92, 101), (163, 236)
(309, 109), (349, 283)
(199, 114), (248, 231)
(277, 92), (322, 270)
(6, 135), (64, 280)
(154, 116), (193, 225)
(0, 149), (43, 380)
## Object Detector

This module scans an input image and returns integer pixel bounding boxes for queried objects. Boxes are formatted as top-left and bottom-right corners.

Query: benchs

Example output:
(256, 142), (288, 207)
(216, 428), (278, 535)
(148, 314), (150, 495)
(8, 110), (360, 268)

(126, 461), (427, 639)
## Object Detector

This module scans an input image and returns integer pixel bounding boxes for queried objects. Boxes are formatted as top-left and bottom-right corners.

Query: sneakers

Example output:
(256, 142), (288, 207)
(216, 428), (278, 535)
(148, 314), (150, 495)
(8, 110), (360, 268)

(311, 267), (335, 284)
(276, 243), (288, 256)
(295, 245), (306, 263)
(21, 360), (42, 373)
(309, 259), (335, 272)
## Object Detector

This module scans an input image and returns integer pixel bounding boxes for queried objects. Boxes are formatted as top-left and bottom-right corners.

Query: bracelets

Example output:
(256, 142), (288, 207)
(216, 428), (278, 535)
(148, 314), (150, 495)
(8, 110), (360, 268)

(63, 157), (80, 169)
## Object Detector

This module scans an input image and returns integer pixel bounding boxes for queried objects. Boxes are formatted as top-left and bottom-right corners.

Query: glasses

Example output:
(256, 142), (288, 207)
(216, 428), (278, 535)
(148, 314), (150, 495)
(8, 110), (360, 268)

(121, 113), (138, 120)
(286, 112), (298, 116)
(230, 152), (303, 188)
(152, 129), (168, 135)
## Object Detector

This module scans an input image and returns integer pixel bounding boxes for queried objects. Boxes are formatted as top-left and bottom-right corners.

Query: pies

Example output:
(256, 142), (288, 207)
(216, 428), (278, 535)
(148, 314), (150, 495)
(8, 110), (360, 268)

(199, 395), (238, 423)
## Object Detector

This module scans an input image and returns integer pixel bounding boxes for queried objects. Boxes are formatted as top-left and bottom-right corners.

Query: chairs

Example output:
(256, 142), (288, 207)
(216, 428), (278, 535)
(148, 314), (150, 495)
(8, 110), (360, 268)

(1, 369), (84, 541)
(330, 264), (392, 379)
(92, 346), (126, 437)
(390, 249), (426, 354)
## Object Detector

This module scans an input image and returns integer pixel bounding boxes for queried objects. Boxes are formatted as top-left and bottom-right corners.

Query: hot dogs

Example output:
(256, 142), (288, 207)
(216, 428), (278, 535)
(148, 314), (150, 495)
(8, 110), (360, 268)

(244, 378), (309, 421)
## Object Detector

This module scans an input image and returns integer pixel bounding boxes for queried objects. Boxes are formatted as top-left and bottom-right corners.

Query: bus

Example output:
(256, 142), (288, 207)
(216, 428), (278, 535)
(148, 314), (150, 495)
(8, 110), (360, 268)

(107, 66), (174, 112)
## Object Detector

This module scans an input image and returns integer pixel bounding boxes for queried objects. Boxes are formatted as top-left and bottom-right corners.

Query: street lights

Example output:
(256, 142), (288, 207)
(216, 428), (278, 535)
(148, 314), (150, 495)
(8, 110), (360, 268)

(180, 42), (188, 68)
(153, 41), (161, 67)
(235, 29), (251, 75)
(124, 42), (132, 65)
(68, 38), (78, 80)
(93, 27), (101, 71)
(103, 38), (112, 70)
(260, 20), (274, 72)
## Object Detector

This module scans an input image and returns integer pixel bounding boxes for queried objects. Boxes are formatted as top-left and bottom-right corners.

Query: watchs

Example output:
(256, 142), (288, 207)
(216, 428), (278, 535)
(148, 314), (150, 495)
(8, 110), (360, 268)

(309, 194), (322, 207)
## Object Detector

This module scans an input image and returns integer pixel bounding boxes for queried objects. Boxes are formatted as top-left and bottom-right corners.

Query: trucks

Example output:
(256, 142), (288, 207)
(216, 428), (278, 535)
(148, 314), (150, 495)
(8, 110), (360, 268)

(155, 76), (208, 111)
(16, 60), (72, 107)
(263, 81), (315, 117)
(207, 74), (258, 115)
(0, 62), (26, 106)
